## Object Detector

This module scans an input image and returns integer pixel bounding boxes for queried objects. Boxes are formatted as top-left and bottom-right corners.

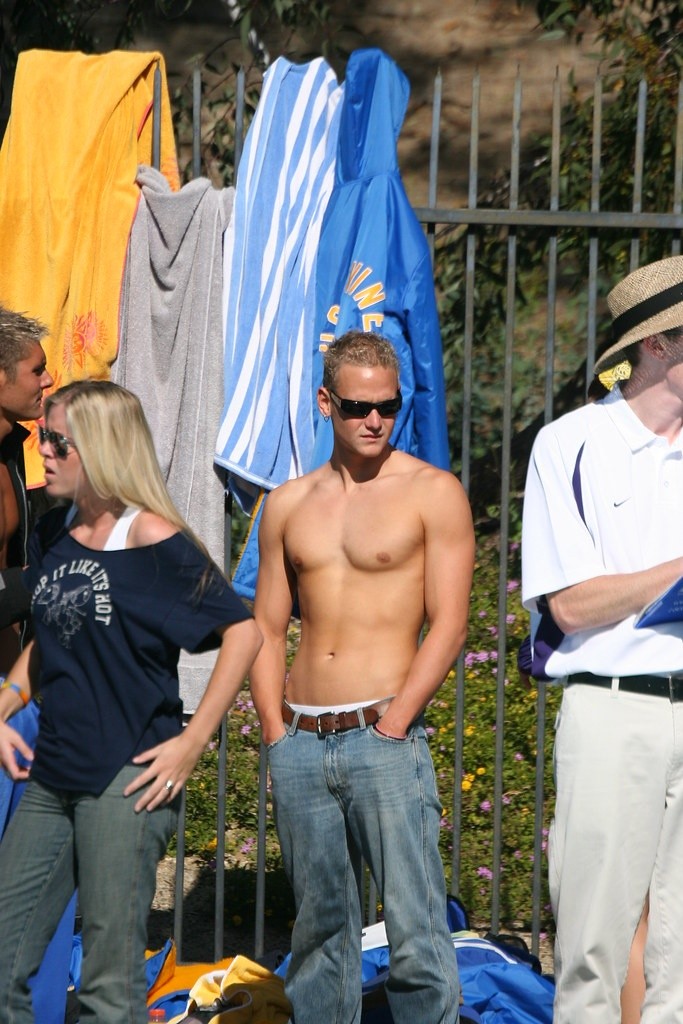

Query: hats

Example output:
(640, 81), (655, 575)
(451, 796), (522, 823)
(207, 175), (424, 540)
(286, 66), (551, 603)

(592, 255), (683, 374)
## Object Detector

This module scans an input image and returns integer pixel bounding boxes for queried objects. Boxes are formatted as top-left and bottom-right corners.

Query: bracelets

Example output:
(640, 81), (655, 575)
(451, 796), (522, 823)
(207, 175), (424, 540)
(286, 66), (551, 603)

(0, 681), (29, 708)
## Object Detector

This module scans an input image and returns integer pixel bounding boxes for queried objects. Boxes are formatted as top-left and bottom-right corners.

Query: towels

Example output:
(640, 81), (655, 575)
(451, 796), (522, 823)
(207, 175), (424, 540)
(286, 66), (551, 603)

(212, 53), (350, 494)
(0, 50), (182, 468)
(189, 949), (294, 1024)
(115, 164), (238, 585)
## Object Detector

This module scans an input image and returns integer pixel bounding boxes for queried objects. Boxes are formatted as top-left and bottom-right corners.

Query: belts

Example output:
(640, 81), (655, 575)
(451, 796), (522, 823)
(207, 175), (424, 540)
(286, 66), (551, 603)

(279, 697), (403, 739)
(567, 673), (683, 703)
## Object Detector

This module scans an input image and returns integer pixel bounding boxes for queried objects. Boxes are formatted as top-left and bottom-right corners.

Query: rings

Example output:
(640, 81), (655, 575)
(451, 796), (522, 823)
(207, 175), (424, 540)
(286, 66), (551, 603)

(165, 782), (174, 789)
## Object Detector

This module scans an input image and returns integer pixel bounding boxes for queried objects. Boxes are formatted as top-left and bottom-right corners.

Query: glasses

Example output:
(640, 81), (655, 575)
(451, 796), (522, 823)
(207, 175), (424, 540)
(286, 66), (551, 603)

(39, 426), (76, 455)
(327, 389), (402, 417)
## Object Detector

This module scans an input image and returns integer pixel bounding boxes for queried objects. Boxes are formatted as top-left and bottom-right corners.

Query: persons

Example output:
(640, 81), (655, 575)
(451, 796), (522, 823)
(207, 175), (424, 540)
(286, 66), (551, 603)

(0, 379), (265, 1024)
(520, 254), (683, 1024)
(0, 305), (74, 1024)
(249, 330), (474, 1024)
